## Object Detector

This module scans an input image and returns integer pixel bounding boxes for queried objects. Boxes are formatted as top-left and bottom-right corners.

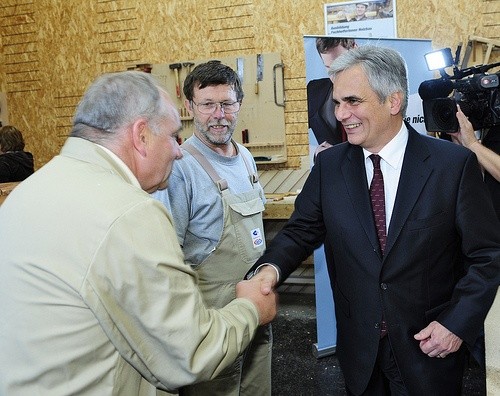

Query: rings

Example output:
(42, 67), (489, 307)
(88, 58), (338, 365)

(439, 353), (446, 358)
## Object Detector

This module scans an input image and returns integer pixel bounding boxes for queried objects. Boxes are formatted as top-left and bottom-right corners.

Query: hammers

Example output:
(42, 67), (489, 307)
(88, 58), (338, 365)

(168, 63), (181, 99)
(183, 62), (195, 75)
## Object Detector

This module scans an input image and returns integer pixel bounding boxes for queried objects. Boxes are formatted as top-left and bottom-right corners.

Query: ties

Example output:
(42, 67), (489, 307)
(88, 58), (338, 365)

(341, 124), (348, 142)
(368, 154), (387, 256)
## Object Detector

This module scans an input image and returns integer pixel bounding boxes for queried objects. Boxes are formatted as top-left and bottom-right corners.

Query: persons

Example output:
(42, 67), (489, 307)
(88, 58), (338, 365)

(0, 73), (276, 396)
(445, 103), (500, 183)
(244, 44), (500, 396)
(307, 37), (358, 165)
(337, 3), (373, 23)
(152, 60), (272, 396)
(0, 126), (34, 184)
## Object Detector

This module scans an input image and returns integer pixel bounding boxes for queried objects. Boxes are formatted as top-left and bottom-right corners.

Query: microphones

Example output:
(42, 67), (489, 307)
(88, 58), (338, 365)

(418, 79), (460, 100)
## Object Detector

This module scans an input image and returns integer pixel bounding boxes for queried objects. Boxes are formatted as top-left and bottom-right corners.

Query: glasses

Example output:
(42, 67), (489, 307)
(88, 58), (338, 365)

(191, 98), (240, 115)
